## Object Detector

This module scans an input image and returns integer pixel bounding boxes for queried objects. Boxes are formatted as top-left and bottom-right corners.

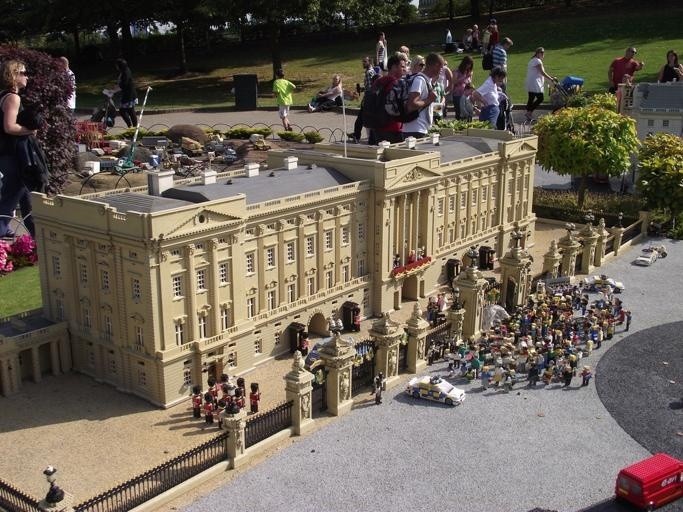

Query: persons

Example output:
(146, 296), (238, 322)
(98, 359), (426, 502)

(525, 47), (558, 124)
(370, 372), (384, 405)
(0, 59), (49, 239)
(108, 58), (139, 128)
(272, 69), (296, 130)
(393, 246), (426, 268)
(488, 253), (494, 270)
(192, 373), (260, 429)
(347, 19), (514, 144)
(608, 46), (644, 95)
(355, 310), (361, 331)
(658, 50), (683, 83)
(308, 74), (344, 112)
(60, 56), (76, 113)
(427, 280), (632, 393)
(302, 340), (308, 355)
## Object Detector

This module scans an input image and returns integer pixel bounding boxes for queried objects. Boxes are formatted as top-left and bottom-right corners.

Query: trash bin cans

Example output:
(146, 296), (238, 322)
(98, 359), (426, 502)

(233, 74), (257, 110)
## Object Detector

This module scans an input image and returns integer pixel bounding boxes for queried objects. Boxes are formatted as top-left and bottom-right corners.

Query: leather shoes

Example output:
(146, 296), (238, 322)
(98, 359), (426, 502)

(525, 113), (536, 121)
(346, 133), (361, 140)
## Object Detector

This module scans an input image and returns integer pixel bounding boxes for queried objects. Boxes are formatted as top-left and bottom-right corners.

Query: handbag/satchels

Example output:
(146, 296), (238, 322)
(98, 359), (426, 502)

(481, 53), (493, 71)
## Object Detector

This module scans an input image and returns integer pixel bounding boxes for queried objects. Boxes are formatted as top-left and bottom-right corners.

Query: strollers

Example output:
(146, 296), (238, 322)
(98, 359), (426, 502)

(548, 76), (589, 115)
(84, 90), (117, 127)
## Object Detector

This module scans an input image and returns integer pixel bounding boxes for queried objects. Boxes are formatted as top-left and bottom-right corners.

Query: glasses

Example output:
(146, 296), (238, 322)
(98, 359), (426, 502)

(631, 50), (637, 55)
(17, 71), (29, 76)
(419, 63), (425, 67)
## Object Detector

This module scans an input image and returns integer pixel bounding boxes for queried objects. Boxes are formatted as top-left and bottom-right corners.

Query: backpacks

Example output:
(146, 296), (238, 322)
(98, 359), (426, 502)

(384, 72), (433, 123)
(355, 84), (385, 126)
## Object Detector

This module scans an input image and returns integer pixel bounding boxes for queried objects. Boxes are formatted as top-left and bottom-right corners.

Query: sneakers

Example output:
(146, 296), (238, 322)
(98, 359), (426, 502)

(0, 227), (20, 240)
